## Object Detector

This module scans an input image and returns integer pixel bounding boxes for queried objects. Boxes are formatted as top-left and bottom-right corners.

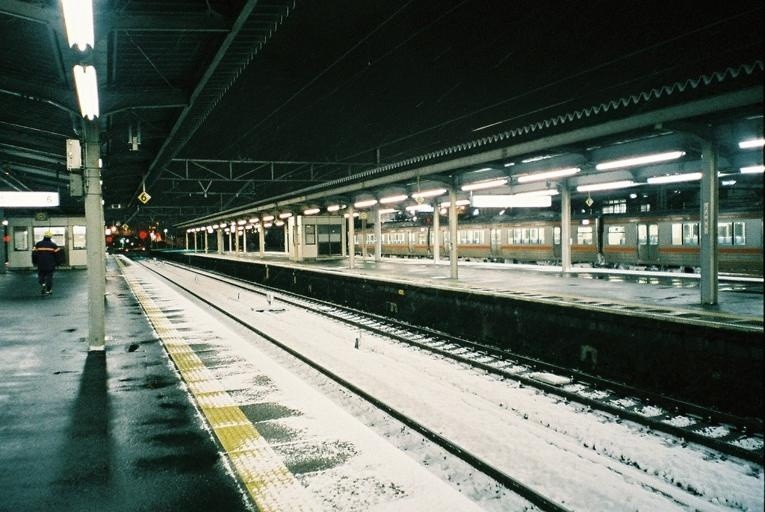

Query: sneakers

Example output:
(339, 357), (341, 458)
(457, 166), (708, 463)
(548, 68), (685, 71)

(40, 283), (52, 294)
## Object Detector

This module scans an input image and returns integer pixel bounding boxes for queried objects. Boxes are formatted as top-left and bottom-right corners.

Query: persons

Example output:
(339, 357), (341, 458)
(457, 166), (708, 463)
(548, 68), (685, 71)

(31, 230), (63, 296)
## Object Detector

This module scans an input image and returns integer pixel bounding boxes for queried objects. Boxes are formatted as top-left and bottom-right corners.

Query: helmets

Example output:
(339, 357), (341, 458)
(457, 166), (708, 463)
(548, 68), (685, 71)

(44, 231), (53, 239)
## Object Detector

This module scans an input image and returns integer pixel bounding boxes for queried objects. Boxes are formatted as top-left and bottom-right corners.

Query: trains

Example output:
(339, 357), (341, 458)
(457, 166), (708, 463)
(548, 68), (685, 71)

(347, 216), (764, 277)
